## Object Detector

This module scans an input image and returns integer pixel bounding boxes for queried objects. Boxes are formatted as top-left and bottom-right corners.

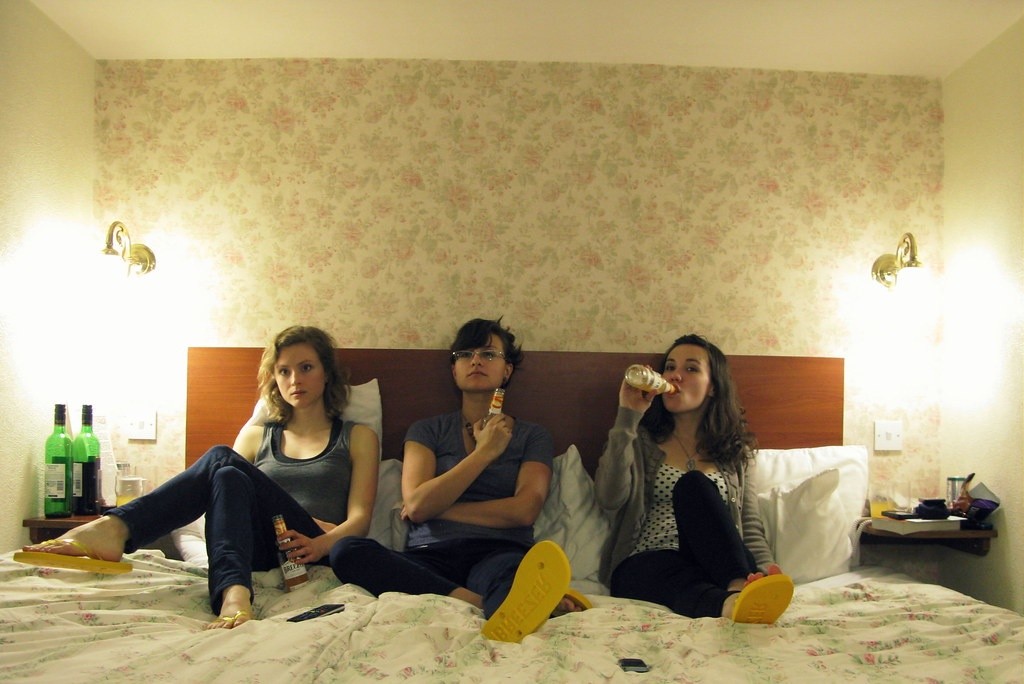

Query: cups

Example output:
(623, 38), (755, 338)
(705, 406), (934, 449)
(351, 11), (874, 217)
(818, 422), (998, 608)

(869, 483), (894, 519)
(116, 477), (143, 509)
(947, 477), (965, 509)
(887, 480), (911, 513)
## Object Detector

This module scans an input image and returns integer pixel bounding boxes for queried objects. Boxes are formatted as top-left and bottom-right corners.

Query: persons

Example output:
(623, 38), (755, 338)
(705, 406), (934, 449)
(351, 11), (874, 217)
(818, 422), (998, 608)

(12, 326), (381, 630)
(330, 318), (593, 641)
(594, 336), (795, 625)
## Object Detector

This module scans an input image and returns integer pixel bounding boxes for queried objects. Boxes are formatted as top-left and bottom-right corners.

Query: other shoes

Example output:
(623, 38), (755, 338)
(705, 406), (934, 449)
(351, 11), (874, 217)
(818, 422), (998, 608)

(552, 589), (591, 619)
(732, 575), (794, 624)
(481, 540), (571, 644)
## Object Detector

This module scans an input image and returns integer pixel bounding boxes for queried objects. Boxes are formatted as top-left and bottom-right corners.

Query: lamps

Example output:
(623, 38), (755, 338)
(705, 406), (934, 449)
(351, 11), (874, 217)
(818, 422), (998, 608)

(99, 221), (155, 277)
(870, 232), (928, 291)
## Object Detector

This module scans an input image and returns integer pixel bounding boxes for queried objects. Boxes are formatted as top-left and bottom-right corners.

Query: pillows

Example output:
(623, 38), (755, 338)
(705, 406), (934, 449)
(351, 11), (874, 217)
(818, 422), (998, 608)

(367, 460), (402, 549)
(252, 377), (382, 463)
(388, 443), (613, 581)
(753, 444), (868, 566)
(757, 467), (853, 582)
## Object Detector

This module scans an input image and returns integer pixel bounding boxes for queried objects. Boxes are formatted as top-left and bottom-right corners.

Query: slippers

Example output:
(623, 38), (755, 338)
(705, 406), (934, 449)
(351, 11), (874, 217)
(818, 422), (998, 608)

(215, 610), (256, 630)
(13, 538), (132, 574)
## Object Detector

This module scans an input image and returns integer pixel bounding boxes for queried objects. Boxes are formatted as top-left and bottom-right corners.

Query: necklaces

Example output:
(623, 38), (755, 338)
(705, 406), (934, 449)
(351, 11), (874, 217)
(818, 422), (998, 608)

(671, 432), (700, 470)
(460, 408), (478, 446)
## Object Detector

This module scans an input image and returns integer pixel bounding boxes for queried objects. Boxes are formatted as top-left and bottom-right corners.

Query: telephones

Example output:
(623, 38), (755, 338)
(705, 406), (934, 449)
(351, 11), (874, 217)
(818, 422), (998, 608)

(890, 490), (922, 513)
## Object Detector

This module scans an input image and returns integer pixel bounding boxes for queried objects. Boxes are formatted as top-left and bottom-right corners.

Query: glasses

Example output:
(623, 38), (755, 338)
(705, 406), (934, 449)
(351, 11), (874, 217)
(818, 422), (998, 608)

(452, 350), (506, 363)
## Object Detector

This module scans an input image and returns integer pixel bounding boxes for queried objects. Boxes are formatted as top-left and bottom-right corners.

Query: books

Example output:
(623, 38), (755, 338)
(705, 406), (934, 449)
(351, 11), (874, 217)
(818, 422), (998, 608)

(871, 516), (960, 535)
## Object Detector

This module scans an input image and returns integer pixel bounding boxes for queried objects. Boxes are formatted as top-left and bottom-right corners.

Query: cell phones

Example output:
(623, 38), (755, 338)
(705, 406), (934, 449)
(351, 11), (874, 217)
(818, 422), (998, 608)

(882, 510), (919, 521)
(288, 604), (345, 623)
(619, 659), (648, 673)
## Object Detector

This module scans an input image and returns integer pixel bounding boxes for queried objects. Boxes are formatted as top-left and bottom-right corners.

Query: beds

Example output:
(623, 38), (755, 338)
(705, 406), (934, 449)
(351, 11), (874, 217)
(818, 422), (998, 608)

(0, 347), (1024, 684)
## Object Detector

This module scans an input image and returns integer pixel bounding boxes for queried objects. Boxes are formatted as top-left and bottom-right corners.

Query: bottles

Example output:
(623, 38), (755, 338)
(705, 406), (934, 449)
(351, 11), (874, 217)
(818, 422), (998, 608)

(624, 364), (676, 395)
(481, 387), (505, 431)
(44, 404), (72, 519)
(272, 514), (309, 592)
(72, 405), (101, 516)
